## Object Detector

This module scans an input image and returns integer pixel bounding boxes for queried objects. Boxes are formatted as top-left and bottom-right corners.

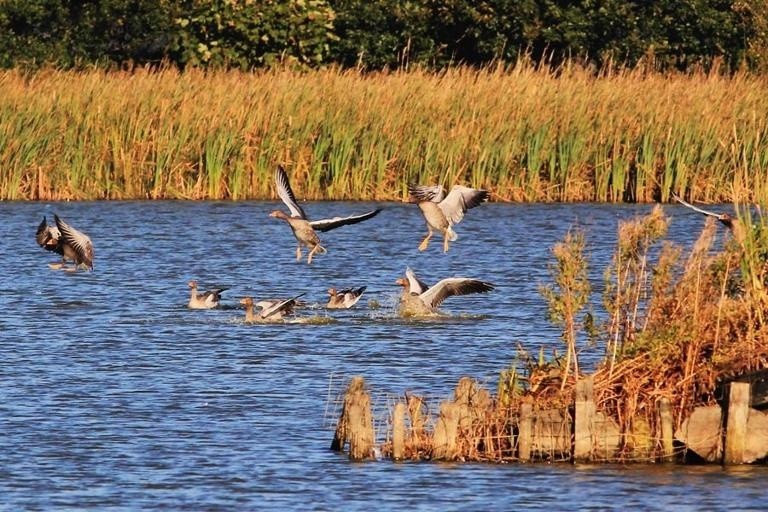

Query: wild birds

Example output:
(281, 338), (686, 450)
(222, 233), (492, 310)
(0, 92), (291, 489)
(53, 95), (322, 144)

(395, 263), (497, 319)
(237, 290), (309, 326)
(188, 280), (231, 310)
(671, 190), (768, 234)
(405, 179), (493, 256)
(34, 212), (95, 275)
(321, 284), (367, 312)
(266, 163), (385, 266)
